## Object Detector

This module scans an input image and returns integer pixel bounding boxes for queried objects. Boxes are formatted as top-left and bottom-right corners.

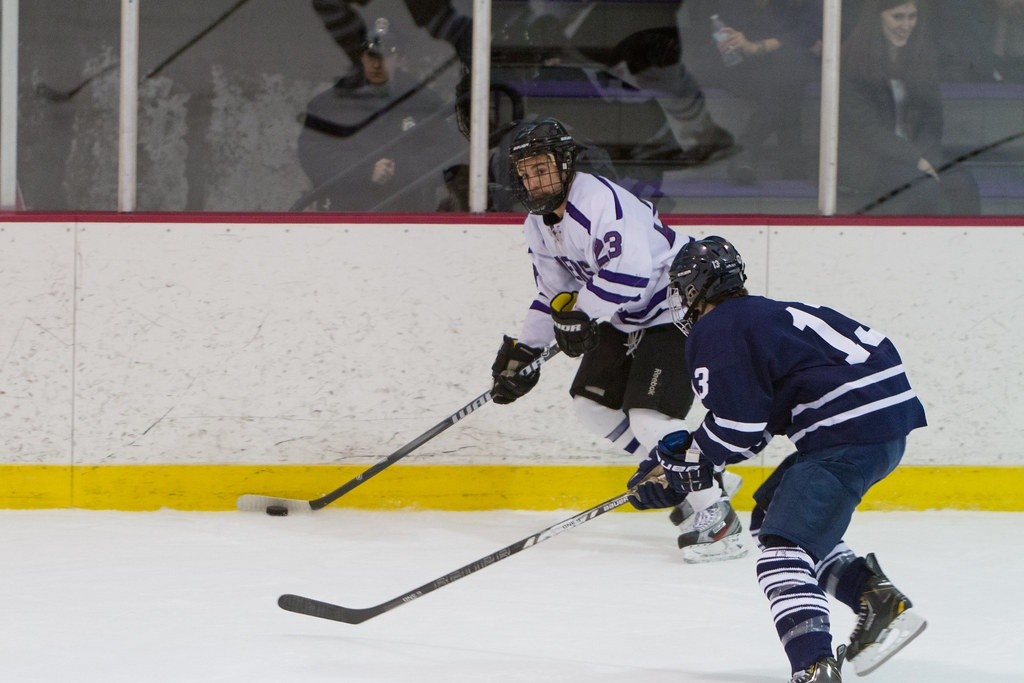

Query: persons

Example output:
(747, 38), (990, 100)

(492, 119), (749, 562)
(628, 236), (926, 683)
(310, 0), (744, 171)
(299, 15), (455, 213)
(838, 0), (980, 216)
(678, 0), (823, 148)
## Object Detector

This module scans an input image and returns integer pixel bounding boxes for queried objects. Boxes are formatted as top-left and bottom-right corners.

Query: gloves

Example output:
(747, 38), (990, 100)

(491, 334), (541, 405)
(627, 430), (714, 510)
(549, 290), (600, 359)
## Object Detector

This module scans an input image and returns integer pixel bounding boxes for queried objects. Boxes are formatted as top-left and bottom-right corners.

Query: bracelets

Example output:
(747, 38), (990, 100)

(924, 166), (932, 172)
(756, 39), (766, 51)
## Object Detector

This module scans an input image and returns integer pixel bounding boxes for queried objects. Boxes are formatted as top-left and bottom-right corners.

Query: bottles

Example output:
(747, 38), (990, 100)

(709, 14), (743, 68)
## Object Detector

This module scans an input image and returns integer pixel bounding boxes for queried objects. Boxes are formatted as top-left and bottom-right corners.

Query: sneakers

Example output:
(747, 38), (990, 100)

(668, 467), (731, 534)
(845, 552), (927, 678)
(785, 644), (847, 683)
(677, 496), (749, 563)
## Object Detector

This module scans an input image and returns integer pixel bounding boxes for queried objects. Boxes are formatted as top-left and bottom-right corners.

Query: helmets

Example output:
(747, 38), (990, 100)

(508, 118), (589, 216)
(666, 235), (747, 337)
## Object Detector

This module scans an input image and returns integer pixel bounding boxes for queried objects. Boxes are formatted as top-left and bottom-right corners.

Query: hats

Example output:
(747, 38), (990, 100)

(355, 16), (409, 57)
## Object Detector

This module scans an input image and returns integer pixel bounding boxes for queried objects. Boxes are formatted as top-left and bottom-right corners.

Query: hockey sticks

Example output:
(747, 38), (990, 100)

(239, 342), (563, 513)
(276, 474), (669, 626)
(848, 129), (1023, 218)
(34, 59), (123, 104)
(135, 2), (246, 83)
(297, 50), (457, 138)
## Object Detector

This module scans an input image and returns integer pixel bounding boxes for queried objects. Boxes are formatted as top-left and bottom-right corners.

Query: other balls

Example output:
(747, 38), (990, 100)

(265, 505), (289, 517)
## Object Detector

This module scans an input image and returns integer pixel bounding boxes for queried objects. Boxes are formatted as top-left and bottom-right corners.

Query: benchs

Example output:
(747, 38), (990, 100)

(487, 77), (1024, 199)
